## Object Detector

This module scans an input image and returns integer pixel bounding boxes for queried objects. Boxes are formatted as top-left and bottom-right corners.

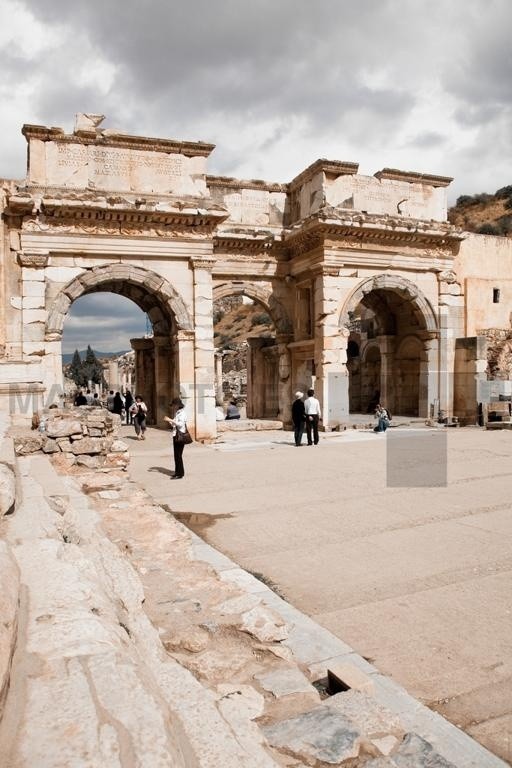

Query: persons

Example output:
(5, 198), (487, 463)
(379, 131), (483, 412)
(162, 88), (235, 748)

(292, 391), (314, 446)
(374, 404), (390, 431)
(304, 390), (322, 445)
(163, 397), (191, 479)
(226, 400), (240, 420)
(74, 388), (147, 441)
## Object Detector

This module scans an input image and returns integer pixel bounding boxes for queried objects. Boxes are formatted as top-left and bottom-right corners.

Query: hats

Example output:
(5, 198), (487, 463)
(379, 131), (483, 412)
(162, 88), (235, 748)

(169, 399), (184, 408)
(135, 394), (143, 401)
(295, 391), (305, 399)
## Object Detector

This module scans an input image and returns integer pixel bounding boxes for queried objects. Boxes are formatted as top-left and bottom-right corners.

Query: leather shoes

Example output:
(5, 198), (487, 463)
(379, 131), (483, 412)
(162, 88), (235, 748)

(171, 474), (182, 479)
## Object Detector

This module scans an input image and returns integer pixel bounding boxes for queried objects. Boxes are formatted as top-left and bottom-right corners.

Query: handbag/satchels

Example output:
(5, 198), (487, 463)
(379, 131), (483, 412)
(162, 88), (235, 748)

(176, 430), (192, 443)
